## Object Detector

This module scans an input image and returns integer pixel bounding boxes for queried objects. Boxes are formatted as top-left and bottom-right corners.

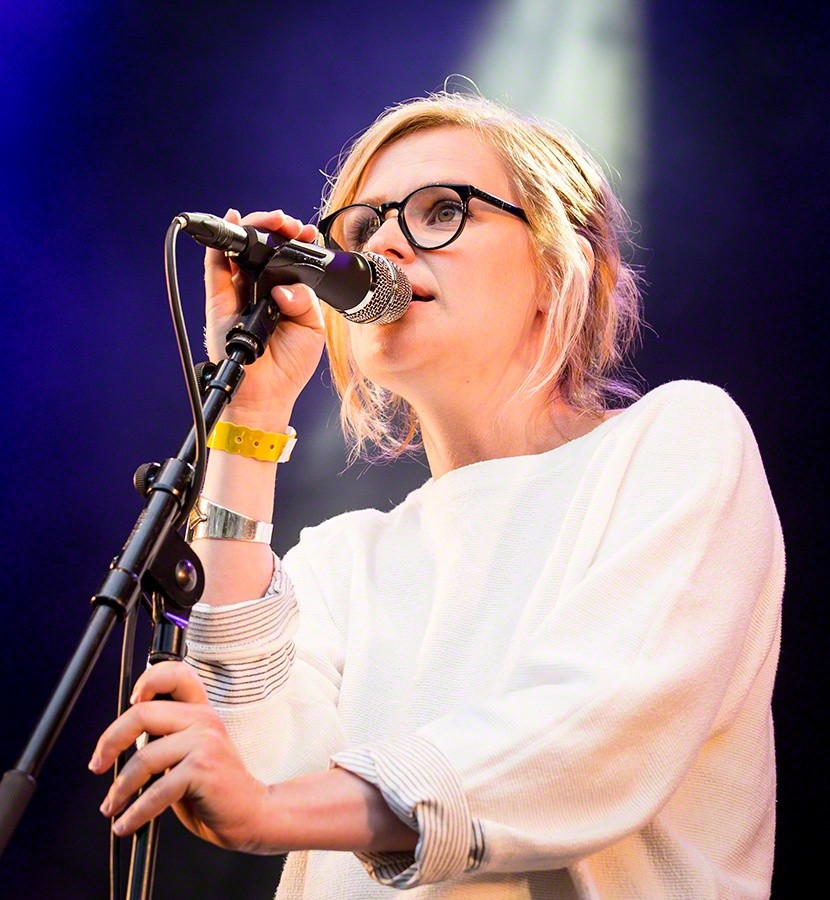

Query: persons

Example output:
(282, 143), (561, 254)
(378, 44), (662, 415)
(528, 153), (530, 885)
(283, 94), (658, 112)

(88, 72), (790, 900)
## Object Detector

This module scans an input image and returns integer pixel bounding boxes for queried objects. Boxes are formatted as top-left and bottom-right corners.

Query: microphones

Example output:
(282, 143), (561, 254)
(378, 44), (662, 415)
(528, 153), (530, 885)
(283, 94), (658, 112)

(179, 212), (412, 325)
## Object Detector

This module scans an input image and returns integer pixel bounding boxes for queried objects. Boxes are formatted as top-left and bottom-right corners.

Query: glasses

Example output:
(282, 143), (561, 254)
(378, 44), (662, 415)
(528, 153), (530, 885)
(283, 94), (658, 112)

(313, 184), (528, 254)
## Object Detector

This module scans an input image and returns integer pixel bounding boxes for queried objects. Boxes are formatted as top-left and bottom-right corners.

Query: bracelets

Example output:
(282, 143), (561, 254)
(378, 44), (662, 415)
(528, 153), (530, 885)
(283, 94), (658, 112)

(182, 494), (273, 545)
(205, 414), (297, 466)
